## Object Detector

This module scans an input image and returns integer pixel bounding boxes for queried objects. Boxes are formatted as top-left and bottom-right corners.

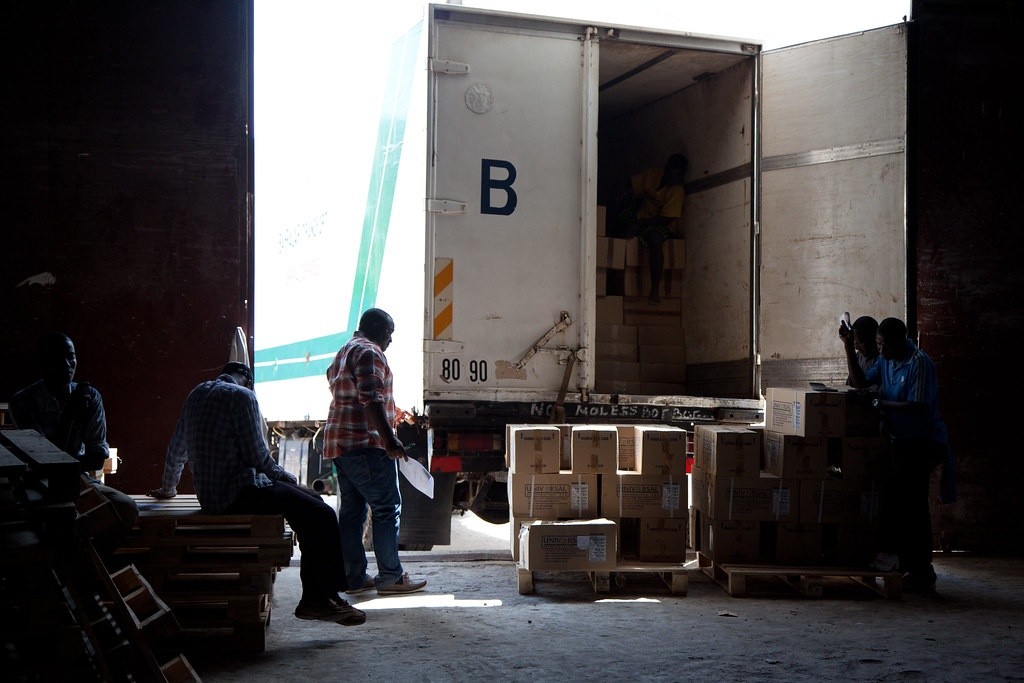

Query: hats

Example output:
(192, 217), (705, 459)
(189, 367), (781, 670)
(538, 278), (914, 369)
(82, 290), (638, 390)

(223, 363), (253, 390)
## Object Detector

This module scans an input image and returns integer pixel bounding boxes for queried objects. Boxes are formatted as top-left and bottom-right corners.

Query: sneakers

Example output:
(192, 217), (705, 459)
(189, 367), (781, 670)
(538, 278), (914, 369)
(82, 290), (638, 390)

(295, 597), (366, 626)
(345, 573), (375, 593)
(377, 573), (427, 594)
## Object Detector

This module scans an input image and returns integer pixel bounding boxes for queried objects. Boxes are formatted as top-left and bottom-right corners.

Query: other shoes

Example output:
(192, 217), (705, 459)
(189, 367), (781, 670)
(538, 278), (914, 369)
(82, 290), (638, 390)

(901, 576), (935, 593)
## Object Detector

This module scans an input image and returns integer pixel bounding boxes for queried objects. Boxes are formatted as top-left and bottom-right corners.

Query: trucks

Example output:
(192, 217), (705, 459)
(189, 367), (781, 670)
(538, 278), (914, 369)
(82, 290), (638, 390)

(253, 4), (910, 551)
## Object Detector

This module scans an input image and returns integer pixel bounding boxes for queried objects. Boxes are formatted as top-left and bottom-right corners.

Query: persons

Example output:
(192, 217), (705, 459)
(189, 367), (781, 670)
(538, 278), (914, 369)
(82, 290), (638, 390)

(4, 333), (110, 501)
(617, 153), (688, 307)
(845, 316), (877, 393)
(322, 310), (428, 596)
(146, 362), (367, 626)
(838, 317), (949, 590)
